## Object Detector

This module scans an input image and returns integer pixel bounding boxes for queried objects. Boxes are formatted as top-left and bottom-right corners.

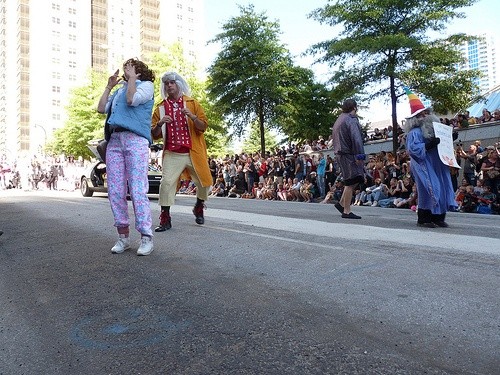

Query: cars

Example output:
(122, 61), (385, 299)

(79, 138), (163, 200)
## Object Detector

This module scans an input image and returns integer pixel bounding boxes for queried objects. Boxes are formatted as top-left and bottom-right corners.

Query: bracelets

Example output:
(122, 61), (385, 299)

(157, 123), (162, 127)
(106, 86), (111, 91)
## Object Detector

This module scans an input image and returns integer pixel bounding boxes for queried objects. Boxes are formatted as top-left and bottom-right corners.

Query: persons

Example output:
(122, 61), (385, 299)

(0, 153), (76, 191)
(151, 71), (213, 231)
(97, 57), (155, 255)
(177, 87), (500, 226)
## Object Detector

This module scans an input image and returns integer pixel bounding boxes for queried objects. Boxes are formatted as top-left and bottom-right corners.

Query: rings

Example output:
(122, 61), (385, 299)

(127, 69), (129, 71)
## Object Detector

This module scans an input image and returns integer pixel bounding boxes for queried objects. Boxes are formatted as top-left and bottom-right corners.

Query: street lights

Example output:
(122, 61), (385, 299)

(34, 123), (47, 162)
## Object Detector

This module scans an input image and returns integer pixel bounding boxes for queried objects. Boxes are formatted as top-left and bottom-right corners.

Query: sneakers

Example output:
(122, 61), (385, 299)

(111, 234), (130, 253)
(136, 237), (154, 256)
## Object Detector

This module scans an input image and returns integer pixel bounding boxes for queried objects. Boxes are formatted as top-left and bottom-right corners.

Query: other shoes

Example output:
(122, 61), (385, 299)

(192, 203), (207, 224)
(334, 202), (362, 219)
(416, 220), (448, 228)
(354, 200), (397, 208)
(155, 217), (172, 231)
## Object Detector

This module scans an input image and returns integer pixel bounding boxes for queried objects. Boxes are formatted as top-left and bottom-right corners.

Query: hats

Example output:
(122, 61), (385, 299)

(485, 146), (495, 150)
(403, 86), (428, 119)
